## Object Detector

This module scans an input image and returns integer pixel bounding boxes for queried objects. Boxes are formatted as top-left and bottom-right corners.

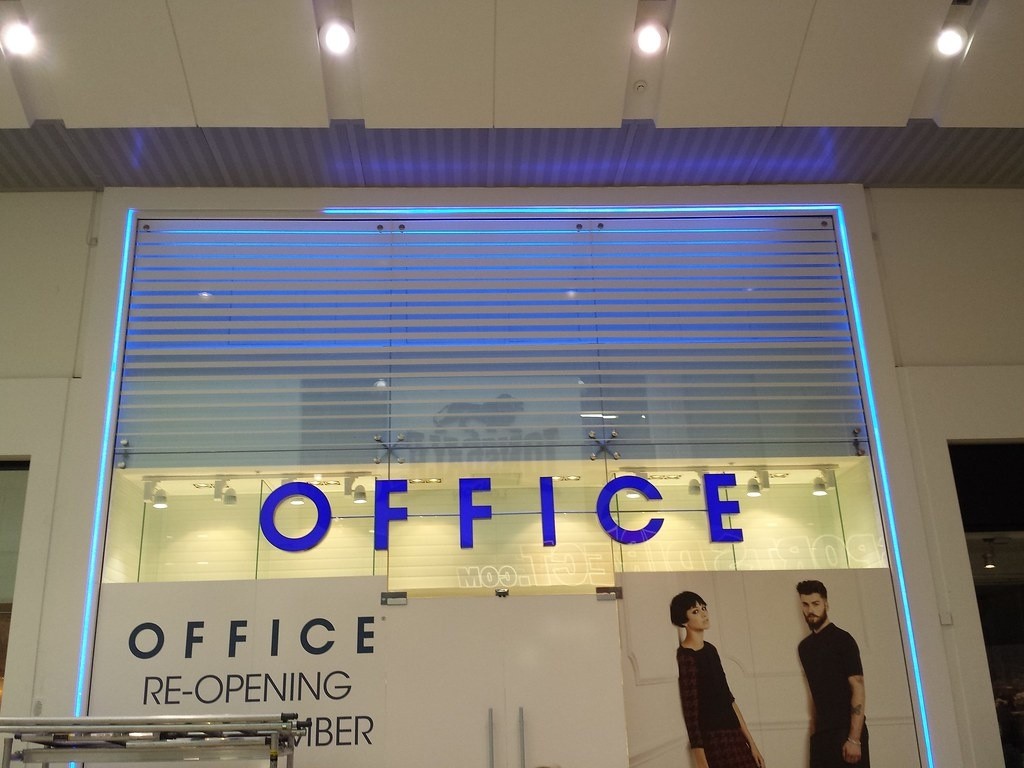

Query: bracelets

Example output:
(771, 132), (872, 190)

(848, 738), (861, 744)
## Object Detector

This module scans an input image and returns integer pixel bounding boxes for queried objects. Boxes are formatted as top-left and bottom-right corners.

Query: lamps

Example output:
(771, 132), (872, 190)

(626, 483), (827, 499)
(984, 552), (997, 568)
(153, 492), (368, 509)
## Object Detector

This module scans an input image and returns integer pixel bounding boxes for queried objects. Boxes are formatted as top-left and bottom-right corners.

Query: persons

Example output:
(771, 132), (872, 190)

(671, 591), (766, 768)
(796, 580), (870, 768)
